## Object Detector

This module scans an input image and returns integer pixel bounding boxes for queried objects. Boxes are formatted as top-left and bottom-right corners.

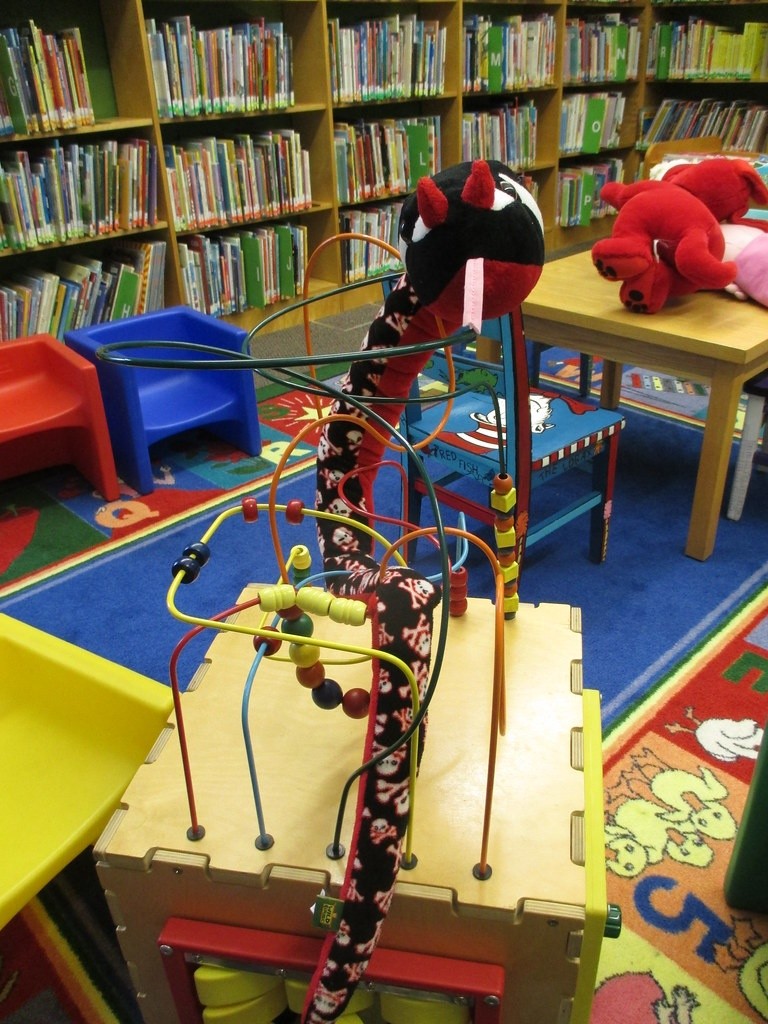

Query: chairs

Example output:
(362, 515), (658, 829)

(396, 317), (626, 573)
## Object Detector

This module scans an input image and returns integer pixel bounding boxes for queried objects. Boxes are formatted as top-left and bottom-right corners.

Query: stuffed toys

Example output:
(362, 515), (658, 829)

(299, 154), (551, 1024)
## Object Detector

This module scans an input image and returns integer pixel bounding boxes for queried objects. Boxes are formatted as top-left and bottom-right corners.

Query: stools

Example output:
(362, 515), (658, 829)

(0, 332), (124, 503)
(65, 305), (263, 494)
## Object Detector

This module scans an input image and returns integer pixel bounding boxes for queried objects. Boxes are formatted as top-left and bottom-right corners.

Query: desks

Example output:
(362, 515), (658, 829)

(518, 249), (768, 564)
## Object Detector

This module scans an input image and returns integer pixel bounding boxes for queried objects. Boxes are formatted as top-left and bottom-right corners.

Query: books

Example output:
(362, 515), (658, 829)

(0, 12), (767, 349)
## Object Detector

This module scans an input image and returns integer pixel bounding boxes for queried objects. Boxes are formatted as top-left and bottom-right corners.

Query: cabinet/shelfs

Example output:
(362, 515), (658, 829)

(1, 0), (768, 336)
